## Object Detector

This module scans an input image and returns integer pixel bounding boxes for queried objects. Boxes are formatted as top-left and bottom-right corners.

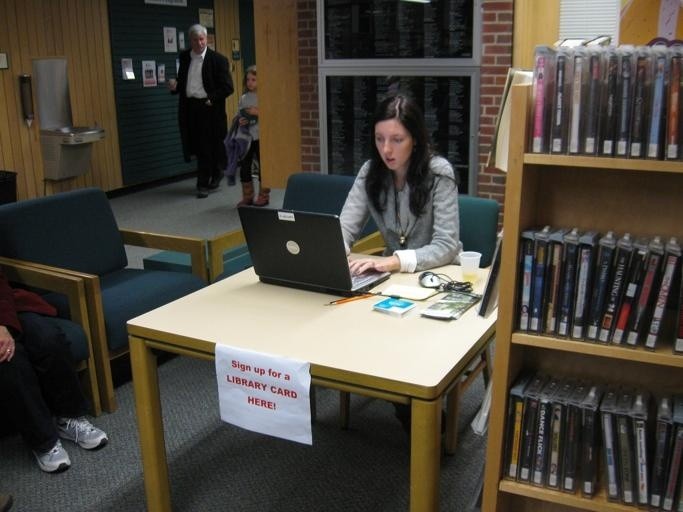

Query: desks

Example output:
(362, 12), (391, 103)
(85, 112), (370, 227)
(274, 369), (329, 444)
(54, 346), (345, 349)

(125, 253), (500, 512)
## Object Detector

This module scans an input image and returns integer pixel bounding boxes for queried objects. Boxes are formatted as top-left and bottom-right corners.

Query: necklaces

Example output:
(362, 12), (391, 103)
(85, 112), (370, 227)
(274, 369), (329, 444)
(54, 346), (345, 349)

(391, 170), (411, 244)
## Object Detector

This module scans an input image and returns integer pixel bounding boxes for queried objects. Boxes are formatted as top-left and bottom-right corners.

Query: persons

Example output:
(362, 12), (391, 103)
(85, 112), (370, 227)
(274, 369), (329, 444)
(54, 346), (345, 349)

(235, 66), (269, 206)
(336, 93), (460, 274)
(168, 24), (234, 199)
(1, 280), (109, 473)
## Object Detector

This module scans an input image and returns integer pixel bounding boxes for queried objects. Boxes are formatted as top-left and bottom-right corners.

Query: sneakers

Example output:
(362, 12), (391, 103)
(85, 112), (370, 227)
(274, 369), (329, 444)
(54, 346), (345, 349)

(32, 438), (71, 473)
(51, 414), (108, 449)
(197, 171), (234, 196)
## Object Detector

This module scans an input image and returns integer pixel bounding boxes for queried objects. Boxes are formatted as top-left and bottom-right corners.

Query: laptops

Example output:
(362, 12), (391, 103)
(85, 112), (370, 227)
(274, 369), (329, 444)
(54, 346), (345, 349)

(237, 205), (392, 297)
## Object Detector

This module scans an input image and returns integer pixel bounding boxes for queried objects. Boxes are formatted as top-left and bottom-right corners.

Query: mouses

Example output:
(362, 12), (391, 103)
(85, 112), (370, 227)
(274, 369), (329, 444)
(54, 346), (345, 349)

(418, 272), (442, 288)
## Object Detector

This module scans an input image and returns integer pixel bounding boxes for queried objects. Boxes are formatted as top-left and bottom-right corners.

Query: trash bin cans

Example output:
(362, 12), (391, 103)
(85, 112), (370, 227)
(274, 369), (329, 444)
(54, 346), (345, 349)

(0, 170), (18, 206)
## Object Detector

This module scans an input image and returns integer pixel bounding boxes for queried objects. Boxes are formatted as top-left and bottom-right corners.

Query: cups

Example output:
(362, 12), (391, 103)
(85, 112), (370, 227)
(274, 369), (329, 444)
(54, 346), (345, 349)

(458, 251), (482, 286)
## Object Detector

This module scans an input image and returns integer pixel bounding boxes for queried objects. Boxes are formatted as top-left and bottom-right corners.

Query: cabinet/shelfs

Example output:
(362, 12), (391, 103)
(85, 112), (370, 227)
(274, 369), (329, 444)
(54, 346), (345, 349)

(479, 79), (683, 511)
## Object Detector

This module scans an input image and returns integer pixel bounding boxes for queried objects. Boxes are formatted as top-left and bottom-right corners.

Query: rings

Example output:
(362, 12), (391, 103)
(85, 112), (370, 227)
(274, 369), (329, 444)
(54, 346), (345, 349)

(6, 349), (13, 354)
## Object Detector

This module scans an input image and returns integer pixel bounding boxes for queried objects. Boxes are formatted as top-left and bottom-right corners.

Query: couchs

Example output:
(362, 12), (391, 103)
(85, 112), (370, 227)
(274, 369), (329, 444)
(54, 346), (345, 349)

(207, 171), (500, 284)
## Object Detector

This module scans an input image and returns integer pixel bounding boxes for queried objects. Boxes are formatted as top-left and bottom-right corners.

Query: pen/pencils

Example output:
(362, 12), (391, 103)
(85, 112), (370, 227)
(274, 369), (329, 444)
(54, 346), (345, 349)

(330, 292), (382, 305)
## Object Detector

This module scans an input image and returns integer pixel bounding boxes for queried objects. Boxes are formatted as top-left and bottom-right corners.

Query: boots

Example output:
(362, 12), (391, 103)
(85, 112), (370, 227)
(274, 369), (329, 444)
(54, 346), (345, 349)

(237, 181), (254, 207)
(254, 181), (270, 206)
